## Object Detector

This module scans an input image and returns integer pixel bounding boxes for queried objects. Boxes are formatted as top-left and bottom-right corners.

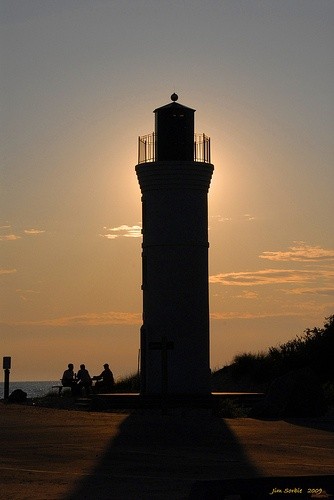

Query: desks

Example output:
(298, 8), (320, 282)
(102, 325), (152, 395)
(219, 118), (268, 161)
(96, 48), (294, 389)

(60, 378), (99, 383)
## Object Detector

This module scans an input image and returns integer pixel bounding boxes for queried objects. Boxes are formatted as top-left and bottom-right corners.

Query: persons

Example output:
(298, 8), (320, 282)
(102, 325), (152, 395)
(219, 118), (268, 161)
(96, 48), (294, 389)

(93, 363), (114, 391)
(76, 364), (92, 394)
(61, 363), (78, 395)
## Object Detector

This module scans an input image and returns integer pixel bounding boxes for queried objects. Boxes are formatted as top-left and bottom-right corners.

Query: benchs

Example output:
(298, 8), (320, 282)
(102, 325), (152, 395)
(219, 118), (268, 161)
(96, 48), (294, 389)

(53, 385), (64, 395)
(91, 390), (140, 417)
(211, 392), (268, 421)
